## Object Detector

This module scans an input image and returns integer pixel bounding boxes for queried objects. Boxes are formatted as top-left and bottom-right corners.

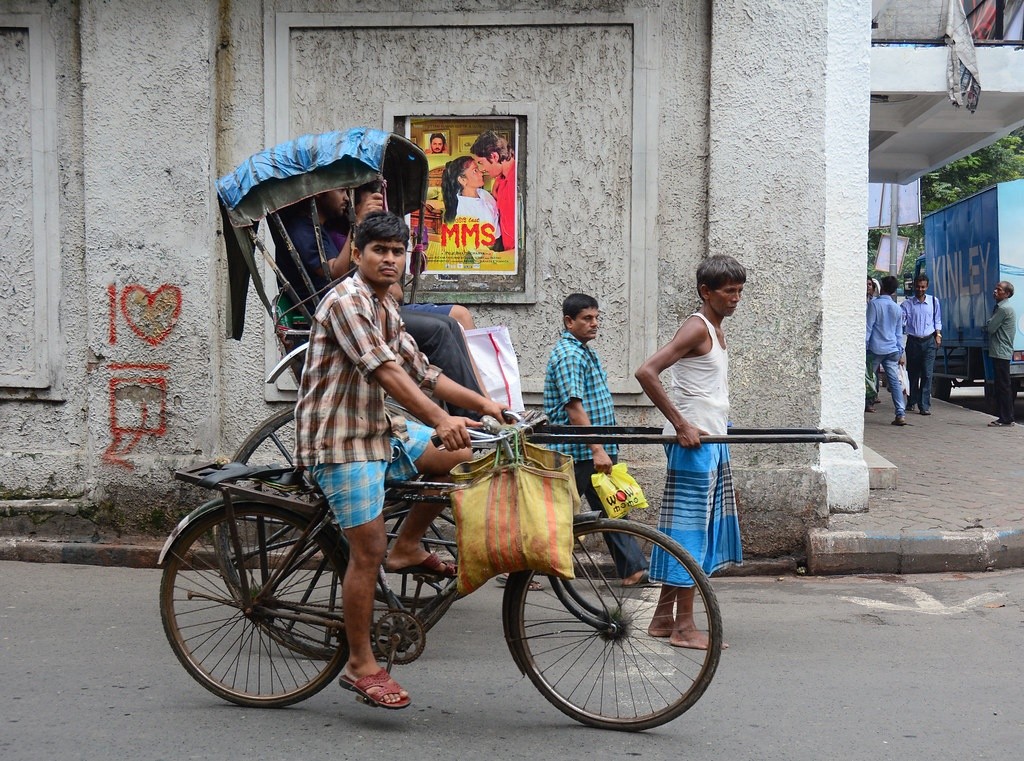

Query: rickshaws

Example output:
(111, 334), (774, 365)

(225, 128), (858, 610)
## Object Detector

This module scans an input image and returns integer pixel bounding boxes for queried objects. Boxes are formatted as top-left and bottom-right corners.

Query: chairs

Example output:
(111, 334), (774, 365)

(271, 289), (307, 387)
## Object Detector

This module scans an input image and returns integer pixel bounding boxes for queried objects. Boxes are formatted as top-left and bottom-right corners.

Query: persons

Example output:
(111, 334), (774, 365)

(441, 155), (501, 253)
(292, 210), (515, 709)
(496, 295), (664, 587)
(424, 133), (448, 154)
(281, 173), (491, 417)
(866, 275), (908, 425)
(985, 281), (1017, 427)
(634, 255), (747, 651)
(470, 130), (515, 250)
(899, 275), (942, 415)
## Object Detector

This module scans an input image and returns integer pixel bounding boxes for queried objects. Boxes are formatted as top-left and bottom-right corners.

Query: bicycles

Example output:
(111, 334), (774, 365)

(158, 407), (724, 732)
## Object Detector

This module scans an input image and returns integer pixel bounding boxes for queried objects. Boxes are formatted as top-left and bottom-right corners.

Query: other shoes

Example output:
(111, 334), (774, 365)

(906, 403), (915, 410)
(891, 417), (906, 425)
(866, 406), (876, 412)
(919, 410), (931, 415)
(988, 420), (1015, 427)
(874, 398), (881, 403)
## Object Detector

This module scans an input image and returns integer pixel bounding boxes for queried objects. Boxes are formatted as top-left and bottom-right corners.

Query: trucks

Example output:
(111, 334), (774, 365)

(904, 177), (1024, 402)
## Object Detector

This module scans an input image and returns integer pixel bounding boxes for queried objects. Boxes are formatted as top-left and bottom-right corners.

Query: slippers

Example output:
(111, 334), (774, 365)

(396, 551), (458, 579)
(339, 667), (412, 710)
(494, 577), (545, 591)
(622, 570), (662, 587)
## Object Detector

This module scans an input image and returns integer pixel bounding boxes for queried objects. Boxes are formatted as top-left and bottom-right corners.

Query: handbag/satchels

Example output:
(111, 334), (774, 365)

(590, 463), (649, 520)
(459, 323), (526, 415)
(448, 427), (585, 594)
(897, 364), (910, 396)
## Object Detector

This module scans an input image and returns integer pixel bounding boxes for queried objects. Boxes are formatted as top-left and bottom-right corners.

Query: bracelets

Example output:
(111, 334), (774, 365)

(937, 334), (941, 337)
